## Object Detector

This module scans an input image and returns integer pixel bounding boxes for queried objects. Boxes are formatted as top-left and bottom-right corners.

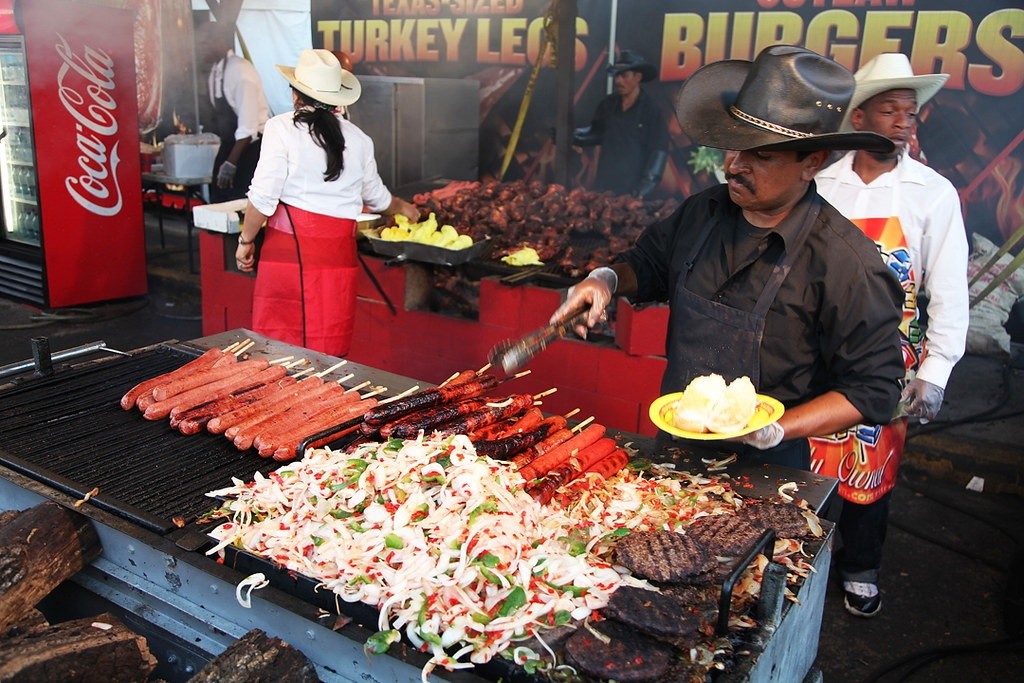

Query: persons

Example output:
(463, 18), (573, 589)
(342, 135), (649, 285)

(235, 48), (422, 359)
(583, 49), (670, 204)
(193, 19), (271, 205)
(546, 44), (907, 474)
(807, 51), (971, 619)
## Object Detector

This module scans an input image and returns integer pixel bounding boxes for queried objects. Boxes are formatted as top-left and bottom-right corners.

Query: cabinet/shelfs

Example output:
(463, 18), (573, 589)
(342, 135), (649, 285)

(346, 73), (480, 192)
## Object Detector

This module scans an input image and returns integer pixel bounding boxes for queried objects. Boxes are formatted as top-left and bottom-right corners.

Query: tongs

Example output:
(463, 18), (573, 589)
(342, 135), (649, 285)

(487, 304), (614, 374)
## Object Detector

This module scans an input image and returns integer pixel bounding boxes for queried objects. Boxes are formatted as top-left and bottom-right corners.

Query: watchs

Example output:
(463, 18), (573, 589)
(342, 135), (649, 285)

(237, 234), (255, 244)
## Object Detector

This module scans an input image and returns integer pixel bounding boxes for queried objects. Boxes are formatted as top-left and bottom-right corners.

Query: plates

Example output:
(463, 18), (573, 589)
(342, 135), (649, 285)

(648, 391), (785, 439)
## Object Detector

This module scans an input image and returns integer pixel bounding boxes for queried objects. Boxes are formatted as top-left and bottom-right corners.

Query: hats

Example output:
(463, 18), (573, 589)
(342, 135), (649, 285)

(676, 45), (895, 154)
(276, 48), (361, 107)
(837, 52), (950, 136)
(605, 49), (659, 83)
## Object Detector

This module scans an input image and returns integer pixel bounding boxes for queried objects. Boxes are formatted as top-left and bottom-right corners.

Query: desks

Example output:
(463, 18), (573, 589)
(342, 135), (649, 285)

(141, 171), (214, 276)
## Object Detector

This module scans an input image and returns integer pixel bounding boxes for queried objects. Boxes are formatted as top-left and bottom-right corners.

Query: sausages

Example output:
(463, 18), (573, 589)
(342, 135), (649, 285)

(122, 347), (628, 503)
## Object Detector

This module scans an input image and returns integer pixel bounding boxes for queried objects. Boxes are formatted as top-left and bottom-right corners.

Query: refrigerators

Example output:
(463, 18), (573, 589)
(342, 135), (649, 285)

(2, 0), (148, 311)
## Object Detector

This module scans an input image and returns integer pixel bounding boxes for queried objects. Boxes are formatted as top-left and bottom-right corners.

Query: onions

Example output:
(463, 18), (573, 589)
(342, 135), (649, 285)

(201, 427), (822, 683)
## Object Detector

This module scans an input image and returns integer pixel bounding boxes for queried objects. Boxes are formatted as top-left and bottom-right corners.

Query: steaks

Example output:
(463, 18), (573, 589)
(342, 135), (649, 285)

(612, 532), (716, 586)
(685, 514), (763, 558)
(565, 619), (678, 682)
(736, 503), (809, 538)
(606, 586), (703, 639)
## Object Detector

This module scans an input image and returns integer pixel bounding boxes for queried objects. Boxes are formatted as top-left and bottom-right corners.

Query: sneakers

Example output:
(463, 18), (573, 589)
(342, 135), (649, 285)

(844, 578), (881, 615)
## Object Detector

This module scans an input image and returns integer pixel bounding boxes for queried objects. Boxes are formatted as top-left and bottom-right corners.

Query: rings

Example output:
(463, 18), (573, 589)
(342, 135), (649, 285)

(237, 260), (243, 271)
